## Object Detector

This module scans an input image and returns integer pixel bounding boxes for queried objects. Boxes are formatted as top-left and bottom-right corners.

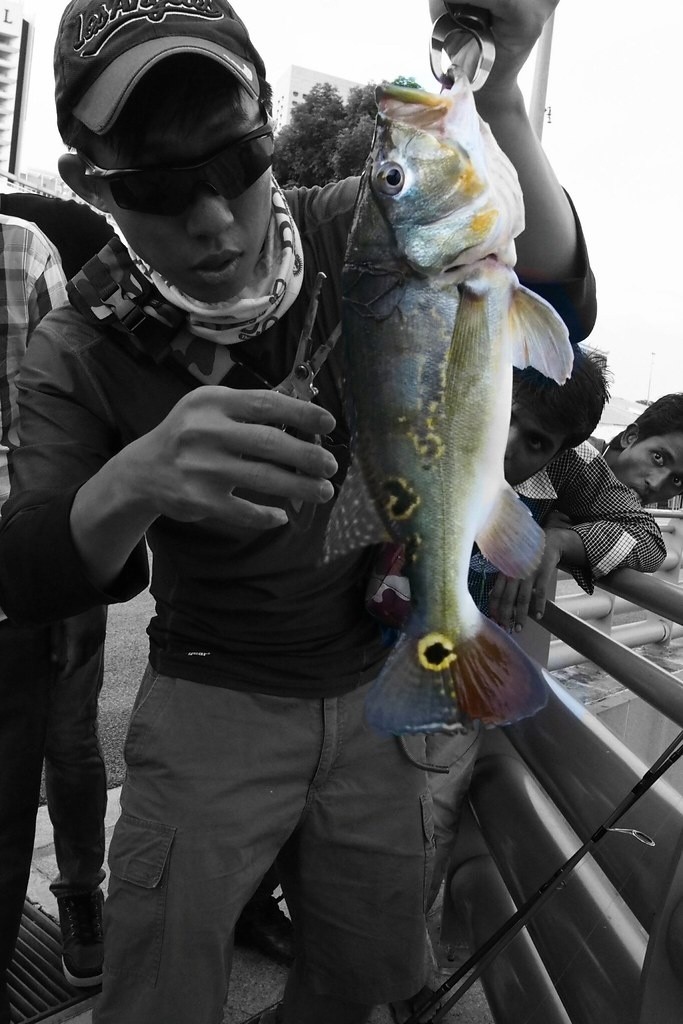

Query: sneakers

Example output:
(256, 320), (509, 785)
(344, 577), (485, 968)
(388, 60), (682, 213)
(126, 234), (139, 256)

(55, 889), (104, 986)
(234, 894), (294, 964)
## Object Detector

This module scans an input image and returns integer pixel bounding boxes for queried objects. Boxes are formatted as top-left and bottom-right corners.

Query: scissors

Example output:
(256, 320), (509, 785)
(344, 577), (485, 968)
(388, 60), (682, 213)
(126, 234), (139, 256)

(239, 270), (343, 530)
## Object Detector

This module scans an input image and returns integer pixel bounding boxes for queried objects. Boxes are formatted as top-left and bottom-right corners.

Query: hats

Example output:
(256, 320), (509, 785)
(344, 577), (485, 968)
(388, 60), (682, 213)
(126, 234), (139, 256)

(53, 0), (260, 134)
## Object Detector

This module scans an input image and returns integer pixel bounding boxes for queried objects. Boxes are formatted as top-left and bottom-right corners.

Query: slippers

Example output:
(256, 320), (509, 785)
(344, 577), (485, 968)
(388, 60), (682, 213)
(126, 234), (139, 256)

(391, 985), (445, 1023)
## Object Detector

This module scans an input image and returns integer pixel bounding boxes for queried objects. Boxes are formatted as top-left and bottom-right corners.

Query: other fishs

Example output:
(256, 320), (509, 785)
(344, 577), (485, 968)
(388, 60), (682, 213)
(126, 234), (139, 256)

(317, 66), (582, 734)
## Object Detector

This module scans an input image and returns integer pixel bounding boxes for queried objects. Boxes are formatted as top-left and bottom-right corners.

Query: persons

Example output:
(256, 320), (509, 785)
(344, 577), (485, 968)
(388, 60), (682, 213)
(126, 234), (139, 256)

(0, 0), (597, 1024)
(1, 79), (683, 990)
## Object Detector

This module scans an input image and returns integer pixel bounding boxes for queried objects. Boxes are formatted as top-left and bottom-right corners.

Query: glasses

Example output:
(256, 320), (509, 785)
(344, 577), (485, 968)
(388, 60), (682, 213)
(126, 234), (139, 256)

(76, 99), (276, 216)
